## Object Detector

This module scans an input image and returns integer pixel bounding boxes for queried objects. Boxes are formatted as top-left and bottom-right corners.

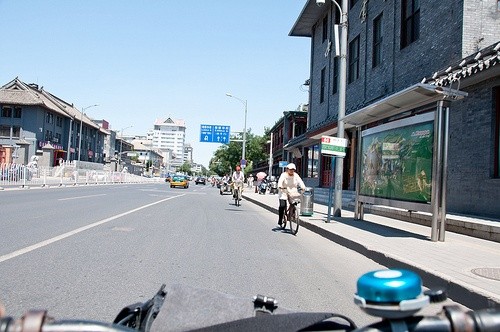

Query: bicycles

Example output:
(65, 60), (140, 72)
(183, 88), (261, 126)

(279, 188), (305, 235)
(232, 181), (243, 207)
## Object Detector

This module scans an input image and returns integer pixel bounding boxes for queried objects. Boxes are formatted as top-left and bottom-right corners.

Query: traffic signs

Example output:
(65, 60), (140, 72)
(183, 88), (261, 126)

(200, 124), (230, 143)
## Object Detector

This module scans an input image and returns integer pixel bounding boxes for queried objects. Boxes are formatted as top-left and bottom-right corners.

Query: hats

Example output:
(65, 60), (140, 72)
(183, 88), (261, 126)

(286, 163), (296, 170)
(236, 166), (240, 171)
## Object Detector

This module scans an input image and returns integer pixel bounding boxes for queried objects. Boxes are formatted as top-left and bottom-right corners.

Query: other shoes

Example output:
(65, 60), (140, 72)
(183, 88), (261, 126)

(239, 199), (242, 201)
(287, 215), (291, 221)
(233, 194), (236, 198)
(278, 221), (282, 225)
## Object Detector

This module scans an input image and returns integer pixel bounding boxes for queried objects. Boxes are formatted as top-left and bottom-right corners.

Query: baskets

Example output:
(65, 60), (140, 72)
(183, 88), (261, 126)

(288, 194), (302, 205)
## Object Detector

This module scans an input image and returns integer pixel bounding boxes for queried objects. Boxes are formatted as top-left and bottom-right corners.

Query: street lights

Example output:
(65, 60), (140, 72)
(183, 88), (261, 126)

(225, 93), (248, 182)
(118, 125), (134, 172)
(77, 105), (99, 169)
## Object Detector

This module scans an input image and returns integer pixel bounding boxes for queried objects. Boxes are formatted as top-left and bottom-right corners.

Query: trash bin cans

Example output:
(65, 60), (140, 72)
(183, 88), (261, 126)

(301, 187), (314, 216)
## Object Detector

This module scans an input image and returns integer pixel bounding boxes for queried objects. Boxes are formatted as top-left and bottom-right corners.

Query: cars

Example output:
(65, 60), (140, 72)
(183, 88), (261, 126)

(208, 174), (233, 194)
(195, 177), (206, 185)
(0, 166), (33, 182)
(165, 173), (192, 189)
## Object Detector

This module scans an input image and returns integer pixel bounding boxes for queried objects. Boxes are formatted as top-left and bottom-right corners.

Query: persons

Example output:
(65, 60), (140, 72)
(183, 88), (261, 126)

(211, 174), (269, 194)
(278, 163), (307, 226)
(231, 166), (244, 201)
